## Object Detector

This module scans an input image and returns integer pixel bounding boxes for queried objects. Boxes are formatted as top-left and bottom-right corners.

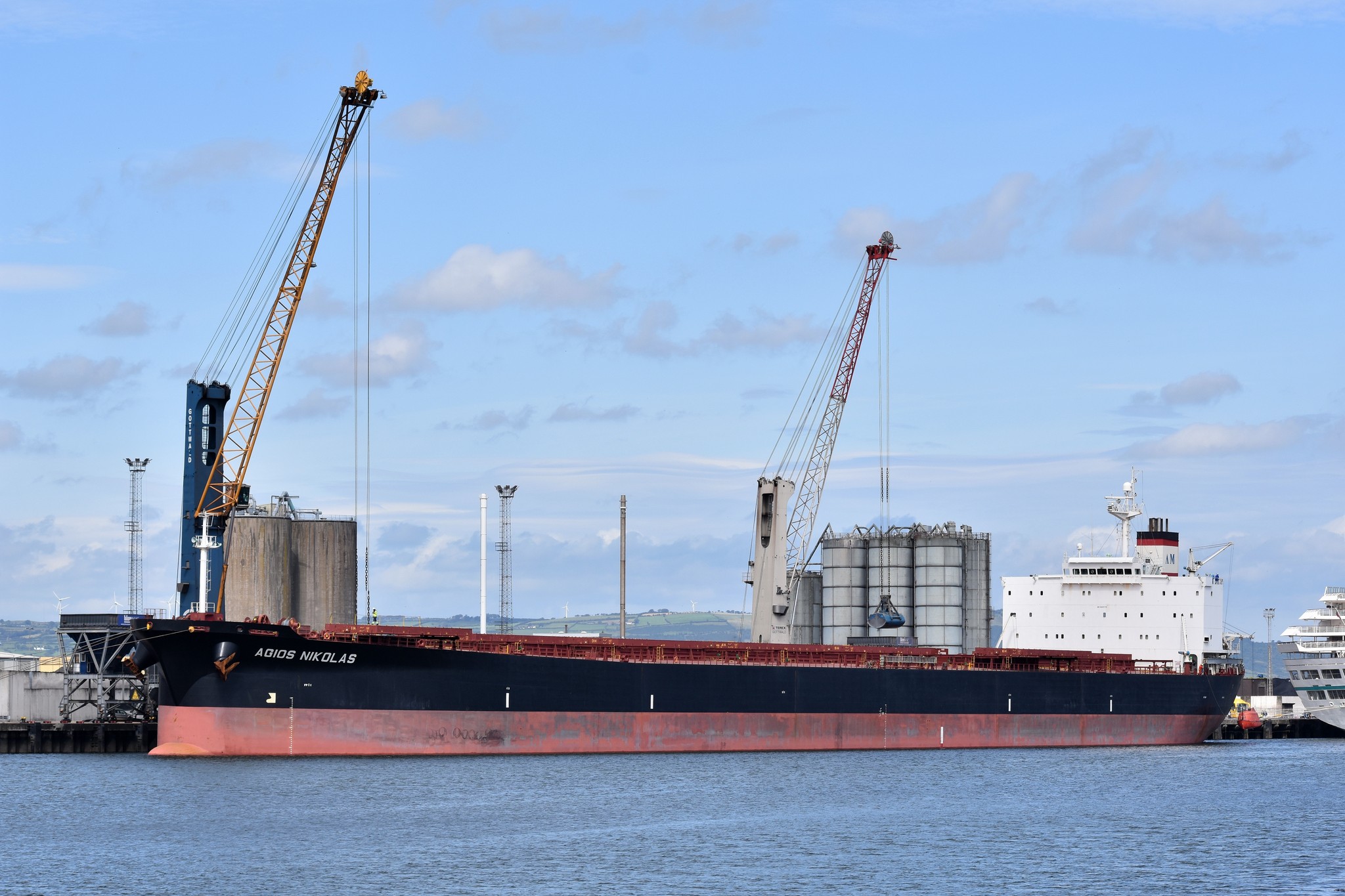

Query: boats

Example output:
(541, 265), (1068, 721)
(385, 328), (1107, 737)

(1276, 588), (1345, 732)
(1236, 707), (1261, 729)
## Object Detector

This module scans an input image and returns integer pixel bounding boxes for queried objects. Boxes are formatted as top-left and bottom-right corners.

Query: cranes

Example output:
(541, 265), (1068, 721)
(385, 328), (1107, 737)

(189, 71), (384, 548)
(741, 232), (898, 636)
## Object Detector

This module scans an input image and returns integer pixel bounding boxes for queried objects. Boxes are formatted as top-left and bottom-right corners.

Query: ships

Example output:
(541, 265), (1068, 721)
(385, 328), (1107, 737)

(136, 479), (1248, 760)
